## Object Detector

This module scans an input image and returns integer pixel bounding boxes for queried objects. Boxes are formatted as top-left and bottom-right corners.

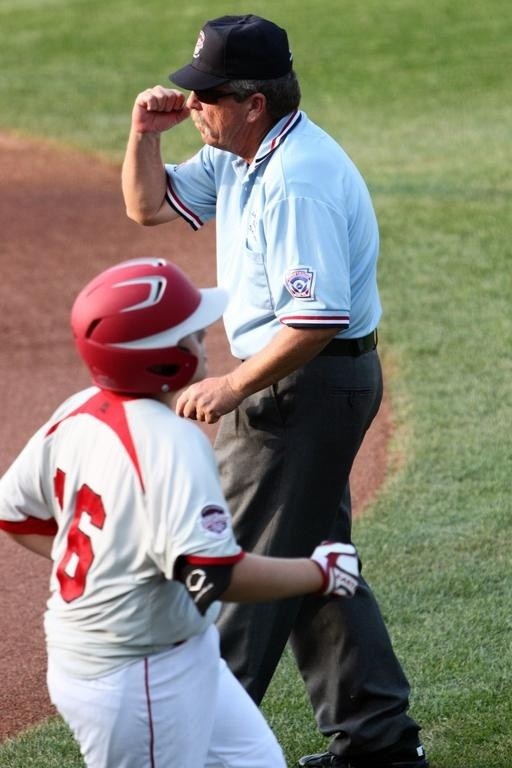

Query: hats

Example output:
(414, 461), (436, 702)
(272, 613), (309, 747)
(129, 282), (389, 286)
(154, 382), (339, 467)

(168, 14), (293, 90)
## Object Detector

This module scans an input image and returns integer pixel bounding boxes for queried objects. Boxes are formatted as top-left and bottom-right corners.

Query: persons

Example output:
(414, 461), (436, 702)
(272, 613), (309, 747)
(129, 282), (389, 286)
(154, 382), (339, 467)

(1, 256), (366, 768)
(120, 15), (426, 767)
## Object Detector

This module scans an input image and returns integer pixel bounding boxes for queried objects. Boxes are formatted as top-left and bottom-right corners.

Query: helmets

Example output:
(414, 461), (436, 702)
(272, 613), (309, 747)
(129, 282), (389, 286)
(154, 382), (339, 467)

(71, 257), (228, 394)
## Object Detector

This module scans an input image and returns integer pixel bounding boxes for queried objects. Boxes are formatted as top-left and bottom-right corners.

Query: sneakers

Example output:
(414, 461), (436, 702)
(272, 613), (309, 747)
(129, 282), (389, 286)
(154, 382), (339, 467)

(298, 744), (429, 767)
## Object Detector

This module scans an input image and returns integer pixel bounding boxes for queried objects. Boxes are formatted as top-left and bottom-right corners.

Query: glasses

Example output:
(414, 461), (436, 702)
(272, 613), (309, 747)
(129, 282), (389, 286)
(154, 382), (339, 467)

(193, 90), (242, 105)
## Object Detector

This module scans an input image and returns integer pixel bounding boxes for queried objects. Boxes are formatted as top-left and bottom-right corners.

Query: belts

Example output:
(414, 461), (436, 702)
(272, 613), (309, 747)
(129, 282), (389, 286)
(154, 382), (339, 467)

(322, 328), (377, 355)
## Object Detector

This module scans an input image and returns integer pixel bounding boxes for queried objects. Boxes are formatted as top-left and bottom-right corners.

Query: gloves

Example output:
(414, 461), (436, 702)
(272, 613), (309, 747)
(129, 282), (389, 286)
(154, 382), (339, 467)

(310, 541), (363, 598)
